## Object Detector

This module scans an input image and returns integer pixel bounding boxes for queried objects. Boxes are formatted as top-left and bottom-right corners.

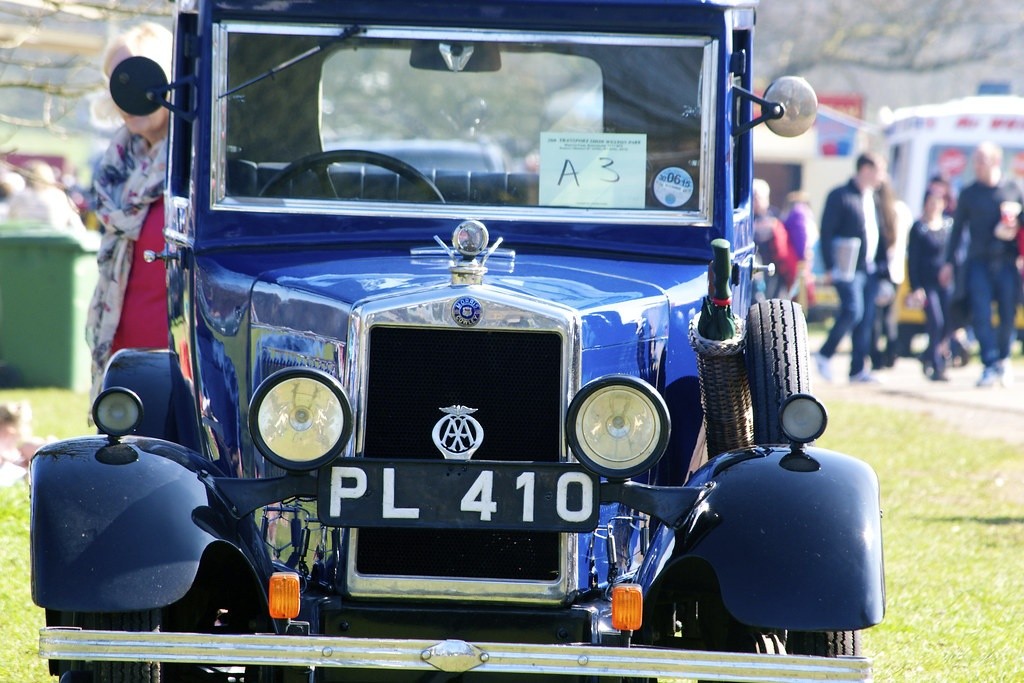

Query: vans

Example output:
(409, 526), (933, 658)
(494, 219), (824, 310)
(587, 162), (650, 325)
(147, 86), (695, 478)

(533, 86), (1024, 348)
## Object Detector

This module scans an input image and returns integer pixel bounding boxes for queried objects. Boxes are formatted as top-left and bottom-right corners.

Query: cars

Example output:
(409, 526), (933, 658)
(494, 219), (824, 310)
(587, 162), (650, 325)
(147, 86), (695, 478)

(320, 98), (528, 178)
(29, 0), (887, 683)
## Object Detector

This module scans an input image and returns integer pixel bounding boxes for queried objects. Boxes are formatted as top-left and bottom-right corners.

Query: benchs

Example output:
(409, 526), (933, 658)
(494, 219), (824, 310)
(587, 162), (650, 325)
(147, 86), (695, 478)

(228, 148), (701, 208)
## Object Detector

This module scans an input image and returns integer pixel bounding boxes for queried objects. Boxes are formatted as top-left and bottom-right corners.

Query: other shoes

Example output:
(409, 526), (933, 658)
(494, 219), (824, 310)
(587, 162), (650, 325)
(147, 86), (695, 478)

(814, 351), (831, 378)
(849, 372), (877, 383)
(976, 365), (1005, 386)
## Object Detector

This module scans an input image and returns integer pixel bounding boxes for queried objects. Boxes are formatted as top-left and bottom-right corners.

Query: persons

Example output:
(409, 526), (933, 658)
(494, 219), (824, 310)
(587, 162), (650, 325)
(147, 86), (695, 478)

(753, 140), (1024, 387)
(82, 23), (173, 436)
(0, 159), (81, 228)
(0, 401), (61, 487)
